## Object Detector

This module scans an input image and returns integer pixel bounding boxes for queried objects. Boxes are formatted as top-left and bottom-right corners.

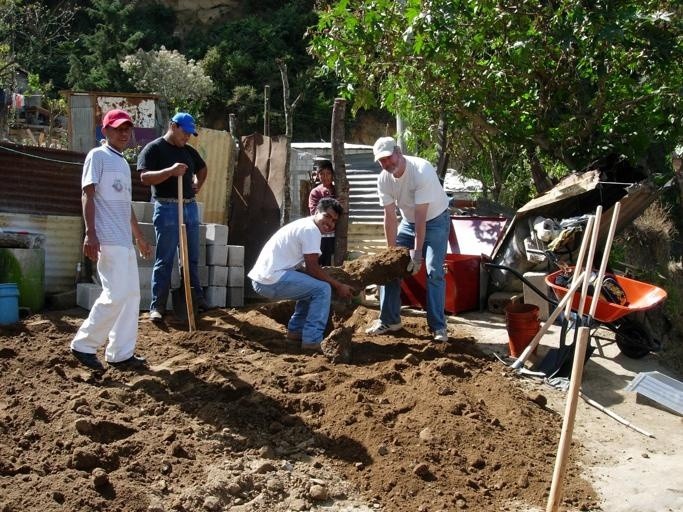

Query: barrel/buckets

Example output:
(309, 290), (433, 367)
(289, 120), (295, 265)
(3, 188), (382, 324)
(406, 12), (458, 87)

(0, 283), (20, 325)
(503, 304), (541, 357)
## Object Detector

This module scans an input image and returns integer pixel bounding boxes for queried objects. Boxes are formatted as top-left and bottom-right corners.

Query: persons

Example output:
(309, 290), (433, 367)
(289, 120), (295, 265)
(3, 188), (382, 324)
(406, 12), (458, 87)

(247, 197), (355, 349)
(365, 136), (450, 343)
(70, 110), (152, 369)
(136, 112), (207, 322)
(309, 161), (339, 264)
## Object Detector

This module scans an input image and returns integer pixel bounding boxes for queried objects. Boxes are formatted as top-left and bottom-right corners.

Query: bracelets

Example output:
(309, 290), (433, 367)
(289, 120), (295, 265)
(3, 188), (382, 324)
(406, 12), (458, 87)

(136, 236), (145, 242)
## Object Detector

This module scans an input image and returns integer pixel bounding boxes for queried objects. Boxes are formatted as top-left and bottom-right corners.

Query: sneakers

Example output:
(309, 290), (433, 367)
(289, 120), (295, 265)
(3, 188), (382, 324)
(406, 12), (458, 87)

(69, 347), (102, 369)
(285, 332), (321, 351)
(365, 317), (402, 336)
(532, 216), (561, 243)
(107, 355), (146, 367)
(433, 329), (449, 343)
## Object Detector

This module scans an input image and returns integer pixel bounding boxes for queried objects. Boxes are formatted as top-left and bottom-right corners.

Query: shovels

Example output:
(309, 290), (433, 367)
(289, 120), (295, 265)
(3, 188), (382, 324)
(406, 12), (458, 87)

(531, 202), (622, 380)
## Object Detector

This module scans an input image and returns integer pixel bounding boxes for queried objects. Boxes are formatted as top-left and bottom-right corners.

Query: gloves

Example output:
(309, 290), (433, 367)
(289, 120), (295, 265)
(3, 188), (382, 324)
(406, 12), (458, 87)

(407, 249), (423, 276)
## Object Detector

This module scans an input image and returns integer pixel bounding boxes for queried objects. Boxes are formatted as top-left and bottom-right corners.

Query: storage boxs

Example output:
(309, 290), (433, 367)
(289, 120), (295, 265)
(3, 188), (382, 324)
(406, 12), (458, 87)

(400, 254), (482, 314)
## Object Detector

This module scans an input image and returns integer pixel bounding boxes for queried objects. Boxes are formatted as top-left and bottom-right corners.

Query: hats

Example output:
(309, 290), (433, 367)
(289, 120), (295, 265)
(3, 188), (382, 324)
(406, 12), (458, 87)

(103, 109), (134, 130)
(172, 112), (198, 137)
(372, 137), (397, 163)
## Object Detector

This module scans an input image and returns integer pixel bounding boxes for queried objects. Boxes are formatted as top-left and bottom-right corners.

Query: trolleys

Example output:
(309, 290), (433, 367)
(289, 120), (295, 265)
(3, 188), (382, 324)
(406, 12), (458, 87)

(481, 248), (667, 359)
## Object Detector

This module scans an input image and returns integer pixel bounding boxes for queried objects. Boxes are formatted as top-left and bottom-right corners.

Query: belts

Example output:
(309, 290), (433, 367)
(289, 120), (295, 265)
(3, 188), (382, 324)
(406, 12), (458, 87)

(155, 197), (196, 203)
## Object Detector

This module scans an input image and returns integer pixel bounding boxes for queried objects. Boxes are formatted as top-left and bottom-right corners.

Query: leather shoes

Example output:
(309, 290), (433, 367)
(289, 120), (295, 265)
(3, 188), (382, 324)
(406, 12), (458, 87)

(149, 310), (164, 322)
(198, 300), (219, 311)
